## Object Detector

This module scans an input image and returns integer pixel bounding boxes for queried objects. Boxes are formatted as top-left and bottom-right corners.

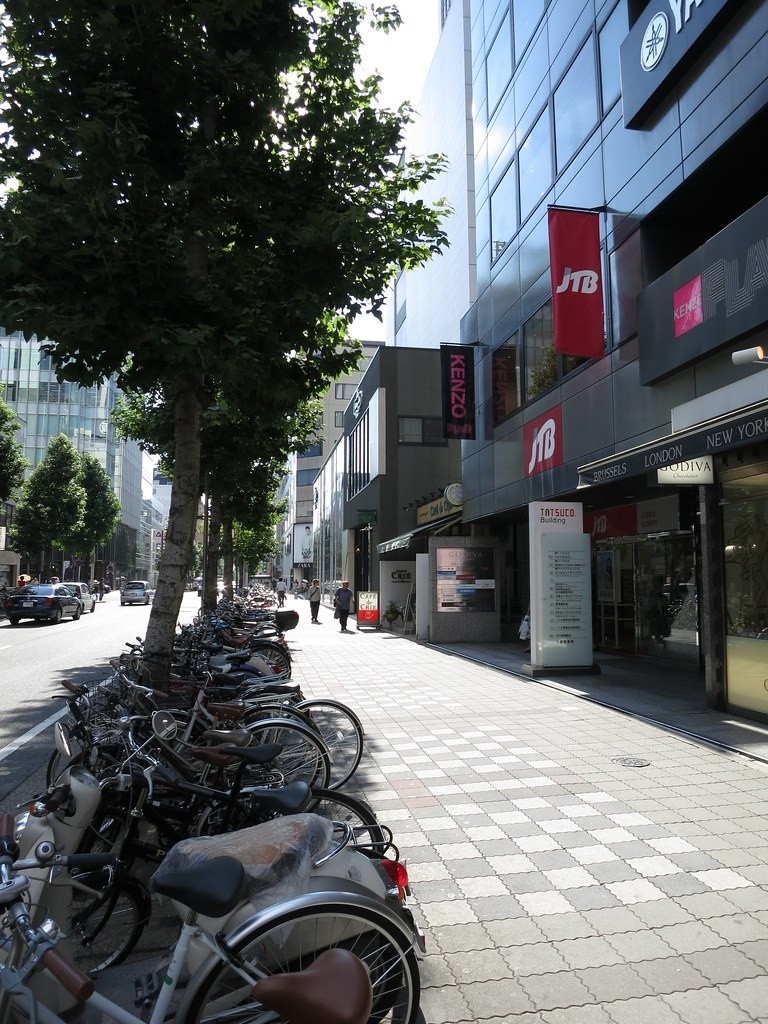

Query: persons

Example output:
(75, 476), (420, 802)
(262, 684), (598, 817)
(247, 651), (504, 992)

(266, 577), (306, 608)
(335, 581), (355, 633)
(309, 579), (321, 622)
(0, 575), (58, 592)
(99, 578), (104, 601)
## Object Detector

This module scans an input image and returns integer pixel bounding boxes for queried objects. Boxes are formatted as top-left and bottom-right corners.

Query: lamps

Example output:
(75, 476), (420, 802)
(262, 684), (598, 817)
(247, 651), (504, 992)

(731, 346), (768, 364)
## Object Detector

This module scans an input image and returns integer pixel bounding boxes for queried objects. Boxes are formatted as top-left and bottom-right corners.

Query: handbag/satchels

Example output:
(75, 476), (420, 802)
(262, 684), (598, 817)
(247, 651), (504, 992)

(517, 610), (530, 640)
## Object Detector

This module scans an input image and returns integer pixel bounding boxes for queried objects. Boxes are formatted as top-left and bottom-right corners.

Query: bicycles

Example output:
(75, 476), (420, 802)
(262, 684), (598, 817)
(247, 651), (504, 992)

(1, 585), (426, 1024)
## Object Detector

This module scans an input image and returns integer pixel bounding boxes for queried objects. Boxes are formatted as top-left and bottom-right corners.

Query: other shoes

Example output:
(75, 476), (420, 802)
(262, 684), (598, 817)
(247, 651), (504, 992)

(523, 648), (530, 652)
(341, 627), (347, 631)
(311, 618), (314, 621)
(315, 619), (318, 622)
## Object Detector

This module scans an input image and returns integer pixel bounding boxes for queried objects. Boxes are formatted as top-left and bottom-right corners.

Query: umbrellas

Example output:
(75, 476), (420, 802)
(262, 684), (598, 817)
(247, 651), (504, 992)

(302, 579), (308, 583)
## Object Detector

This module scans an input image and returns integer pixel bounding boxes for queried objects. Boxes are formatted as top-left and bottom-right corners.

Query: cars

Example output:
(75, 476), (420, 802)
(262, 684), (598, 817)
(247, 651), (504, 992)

(4, 583), (82, 626)
(121, 580), (156, 605)
(56, 581), (96, 615)
(88, 580), (111, 594)
(197, 576), (236, 597)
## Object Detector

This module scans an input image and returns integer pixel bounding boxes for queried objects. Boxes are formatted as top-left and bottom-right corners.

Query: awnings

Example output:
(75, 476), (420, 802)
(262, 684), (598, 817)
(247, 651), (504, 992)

(376, 513), (462, 554)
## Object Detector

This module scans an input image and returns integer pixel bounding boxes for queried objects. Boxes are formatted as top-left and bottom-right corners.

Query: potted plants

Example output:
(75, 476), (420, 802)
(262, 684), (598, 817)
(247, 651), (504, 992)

(381, 601), (403, 623)
